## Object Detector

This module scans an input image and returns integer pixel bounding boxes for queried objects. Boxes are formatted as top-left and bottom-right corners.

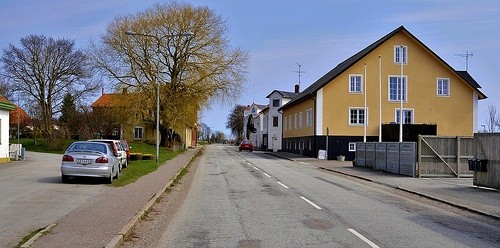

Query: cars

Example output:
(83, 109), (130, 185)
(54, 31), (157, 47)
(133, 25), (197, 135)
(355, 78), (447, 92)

(239, 139), (254, 152)
(60, 139), (131, 183)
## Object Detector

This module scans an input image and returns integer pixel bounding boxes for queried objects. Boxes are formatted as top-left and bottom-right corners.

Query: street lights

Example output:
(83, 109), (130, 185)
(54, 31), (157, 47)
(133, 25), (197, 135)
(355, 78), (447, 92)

(124, 30), (195, 164)
